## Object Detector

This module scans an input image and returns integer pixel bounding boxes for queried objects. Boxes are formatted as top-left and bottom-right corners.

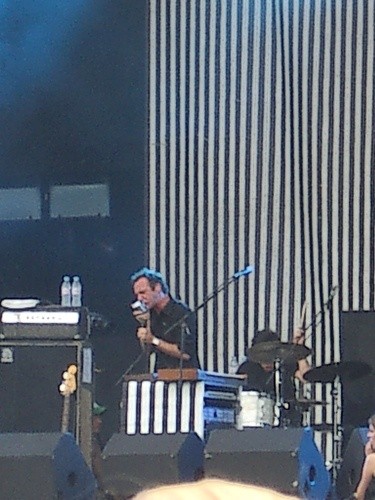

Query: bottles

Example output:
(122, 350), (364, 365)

(71, 276), (81, 306)
(230, 356), (237, 373)
(61, 276), (71, 306)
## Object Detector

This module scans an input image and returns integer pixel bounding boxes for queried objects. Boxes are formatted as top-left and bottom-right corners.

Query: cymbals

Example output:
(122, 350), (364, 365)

(246, 341), (312, 364)
(303, 360), (373, 383)
(285, 398), (330, 406)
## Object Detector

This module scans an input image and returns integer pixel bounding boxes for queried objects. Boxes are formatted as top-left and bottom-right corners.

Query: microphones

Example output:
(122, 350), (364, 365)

(326, 288), (336, 309)
(232, 266), (253, 280)
(139, 318), (148, 353)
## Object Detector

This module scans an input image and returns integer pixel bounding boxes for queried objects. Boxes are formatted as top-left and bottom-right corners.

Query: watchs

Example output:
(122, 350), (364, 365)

(152, 336), (160, 348)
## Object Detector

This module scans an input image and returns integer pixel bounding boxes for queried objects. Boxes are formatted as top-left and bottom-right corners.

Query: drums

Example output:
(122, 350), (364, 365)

(239, 391), (276, 427)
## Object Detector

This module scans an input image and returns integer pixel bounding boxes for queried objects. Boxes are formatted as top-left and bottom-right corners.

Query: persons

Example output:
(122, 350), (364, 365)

(131, 267), (198, 371)
(352, 415), (375, 500)
(236, 328), (310, 401)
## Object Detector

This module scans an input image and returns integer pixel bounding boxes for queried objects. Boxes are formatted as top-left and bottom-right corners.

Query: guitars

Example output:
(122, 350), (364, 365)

(59, 365), (76, 433)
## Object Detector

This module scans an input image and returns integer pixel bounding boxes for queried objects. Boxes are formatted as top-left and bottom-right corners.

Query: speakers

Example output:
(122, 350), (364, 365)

(203, 427), (331, 500)
(0, 432), (99, 500)
(99, 432), (205, 498)
(335, 428), (375, 500)
